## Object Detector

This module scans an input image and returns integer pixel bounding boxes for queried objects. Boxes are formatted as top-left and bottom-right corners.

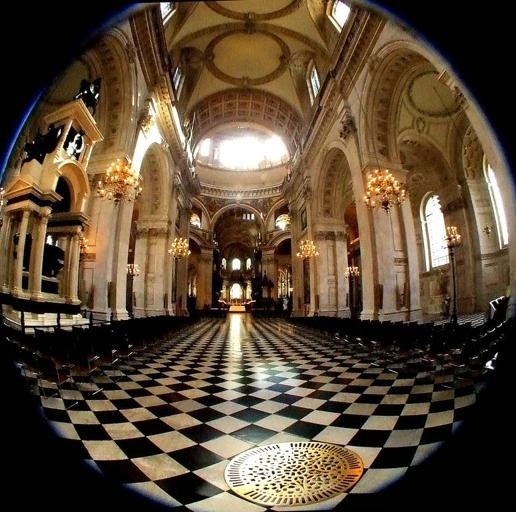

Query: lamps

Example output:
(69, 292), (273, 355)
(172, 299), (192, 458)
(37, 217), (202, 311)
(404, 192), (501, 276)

(361, 168), (407, 214)
(97, 156), (144, 209)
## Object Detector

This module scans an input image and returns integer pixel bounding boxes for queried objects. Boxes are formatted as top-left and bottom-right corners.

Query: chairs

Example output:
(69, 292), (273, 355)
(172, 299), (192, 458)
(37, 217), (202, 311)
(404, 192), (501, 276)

(289, 316), (504, 387)
(6, 315), (193, 411)
